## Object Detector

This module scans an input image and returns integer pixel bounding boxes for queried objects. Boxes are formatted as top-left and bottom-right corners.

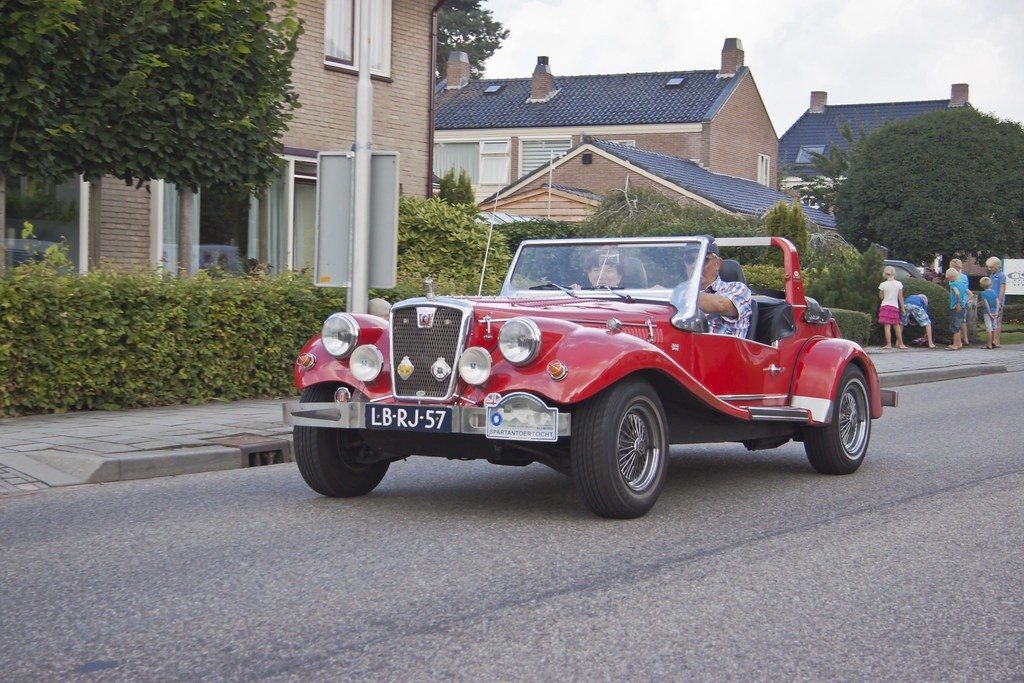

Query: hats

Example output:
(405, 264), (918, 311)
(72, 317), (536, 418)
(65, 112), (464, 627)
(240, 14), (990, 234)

(679, 234), (720, 256)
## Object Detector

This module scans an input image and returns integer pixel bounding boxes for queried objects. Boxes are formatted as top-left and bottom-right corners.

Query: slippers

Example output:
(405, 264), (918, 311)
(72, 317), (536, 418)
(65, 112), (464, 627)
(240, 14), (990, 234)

(882, 345), (891, 349)
(899, 345), (909, 349)
(993, 341), (1001, 348)
(980, 345), (993, 349)
(944, 343), (962, 351)
(929, 344), (939, 349)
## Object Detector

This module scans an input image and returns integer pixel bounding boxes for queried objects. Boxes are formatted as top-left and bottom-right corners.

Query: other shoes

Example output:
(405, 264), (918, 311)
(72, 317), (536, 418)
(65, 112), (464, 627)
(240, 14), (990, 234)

(962, 342), (970, 346)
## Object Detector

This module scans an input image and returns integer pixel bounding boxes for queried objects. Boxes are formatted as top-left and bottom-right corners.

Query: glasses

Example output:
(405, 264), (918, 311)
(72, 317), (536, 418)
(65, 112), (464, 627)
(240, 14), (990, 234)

(683, 253), (716, 265)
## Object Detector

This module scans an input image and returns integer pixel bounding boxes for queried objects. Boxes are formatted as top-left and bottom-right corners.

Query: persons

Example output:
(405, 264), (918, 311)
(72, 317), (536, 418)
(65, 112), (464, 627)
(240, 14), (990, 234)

(568, 248), (638, 290)
(896, 294), (937, 349)
(944, 256), (1006, 350)
(652, 235), (752, 339)
(877, 266), (908, 349)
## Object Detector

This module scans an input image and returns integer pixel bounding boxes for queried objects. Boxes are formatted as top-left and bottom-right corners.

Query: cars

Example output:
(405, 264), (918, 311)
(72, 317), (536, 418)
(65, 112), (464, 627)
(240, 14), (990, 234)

(884, 260), (922, 278)
(282, 234), (898, 520)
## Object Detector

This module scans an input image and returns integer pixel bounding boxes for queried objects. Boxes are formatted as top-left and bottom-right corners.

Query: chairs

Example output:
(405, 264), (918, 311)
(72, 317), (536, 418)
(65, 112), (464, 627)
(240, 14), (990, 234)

(718, 260), (760, 340)
(619, 258), (647, 290)
